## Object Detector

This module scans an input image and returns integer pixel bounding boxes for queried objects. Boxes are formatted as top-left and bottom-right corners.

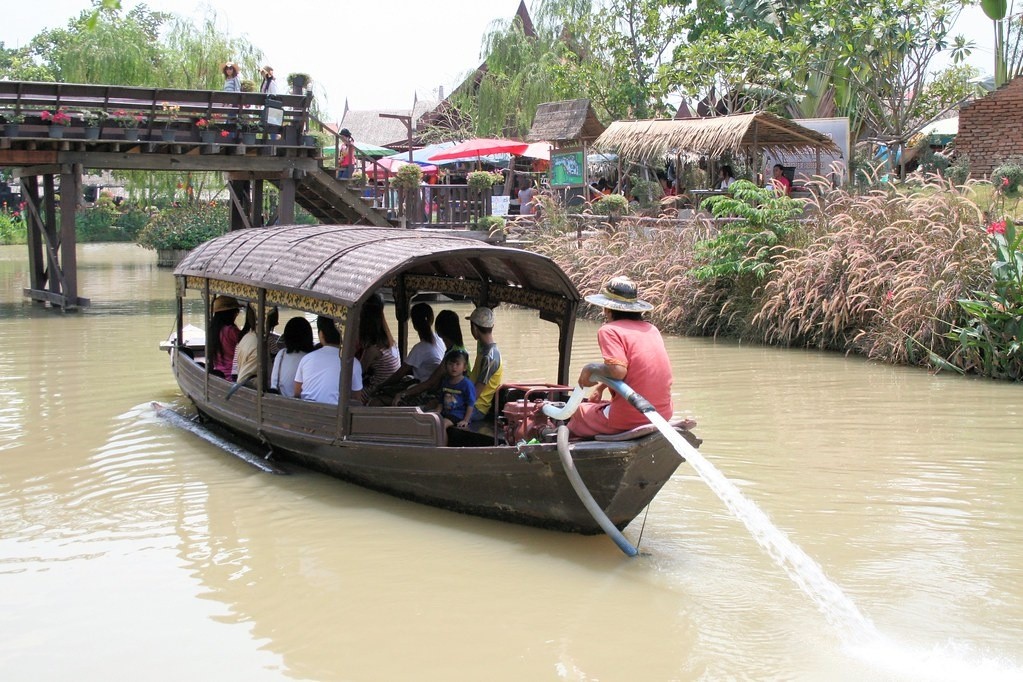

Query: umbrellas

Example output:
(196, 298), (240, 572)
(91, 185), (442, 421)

(363, 159), (437, 178)
(382, 141), (487, 167)
(319, 142), (400, 158)
(427, 138), (529, 173)
(521, 140), (561, 160)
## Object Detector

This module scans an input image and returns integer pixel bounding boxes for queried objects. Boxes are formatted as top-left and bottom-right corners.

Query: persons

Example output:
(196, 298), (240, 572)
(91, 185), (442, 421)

(255, 66), (281, 140)
(294, 313), (363, 406)
(220, 60), (242, 125)
(770, 163), (790, 199)
(430, 349), (477, 446)
(355, 291), (471, 406)
(517, 178), (539, 214)
(655, 172), (678, 197)
(337, 128), (356, 179)
(590, 177), (610, 203)
(719, 165), (736, 191)
(211, 295), (313, 395)
(565, 275), (672, 439)
(366, 175), (432, 208)
(465, 308), (503, 421)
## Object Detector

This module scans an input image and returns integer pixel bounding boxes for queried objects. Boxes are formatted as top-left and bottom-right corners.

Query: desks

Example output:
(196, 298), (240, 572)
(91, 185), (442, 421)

(693, 190), (732, 213)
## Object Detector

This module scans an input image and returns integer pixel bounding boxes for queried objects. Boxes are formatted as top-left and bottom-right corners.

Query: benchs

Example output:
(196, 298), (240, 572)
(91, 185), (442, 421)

(448, 415), (506, 448)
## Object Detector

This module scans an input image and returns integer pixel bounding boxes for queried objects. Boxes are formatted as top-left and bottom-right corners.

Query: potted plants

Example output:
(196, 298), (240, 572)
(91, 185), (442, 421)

(595, 193), (629, 216)
(240, 79), (255, 92)
(477, 215), (507, 243)
(351, 172), (370, 188)
(302, 130), (332, 148)
(629, 180), (662, 203)
(287, 73), (311, 94)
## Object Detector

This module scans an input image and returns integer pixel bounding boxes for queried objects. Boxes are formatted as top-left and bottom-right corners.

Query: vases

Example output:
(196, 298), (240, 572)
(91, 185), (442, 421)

(493, 185), (504, 196)
(4, 123), (256, 144)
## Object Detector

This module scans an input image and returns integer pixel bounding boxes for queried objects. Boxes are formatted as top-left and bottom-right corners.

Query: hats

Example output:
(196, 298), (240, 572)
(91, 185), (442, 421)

(258, 65), (275, 80)
(584, 275), (654, 312)
(339, 128), (351, 136)
(465, 307), (495, 327)
(220, 62), (240, 73)
(213, 295), (242, 312)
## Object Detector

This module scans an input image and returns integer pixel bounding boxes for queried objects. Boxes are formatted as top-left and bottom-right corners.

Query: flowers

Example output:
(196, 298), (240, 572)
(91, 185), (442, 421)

(1, 101), (263, 137)
(490, 168), (505, 185)
(465, 170), (493, 189)
(392, 164), (421, 187)
